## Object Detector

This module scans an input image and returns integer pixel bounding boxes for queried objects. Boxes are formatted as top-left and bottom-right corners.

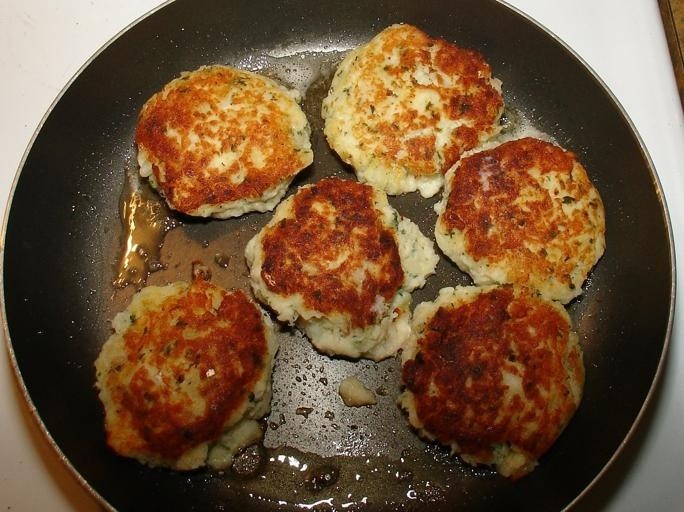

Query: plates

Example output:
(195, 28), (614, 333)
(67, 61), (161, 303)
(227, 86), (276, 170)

(2, 0), (677, 511)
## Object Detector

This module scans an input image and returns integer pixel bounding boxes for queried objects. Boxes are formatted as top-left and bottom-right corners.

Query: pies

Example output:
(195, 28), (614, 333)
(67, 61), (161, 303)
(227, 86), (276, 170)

(93, 26), (606, 483)
